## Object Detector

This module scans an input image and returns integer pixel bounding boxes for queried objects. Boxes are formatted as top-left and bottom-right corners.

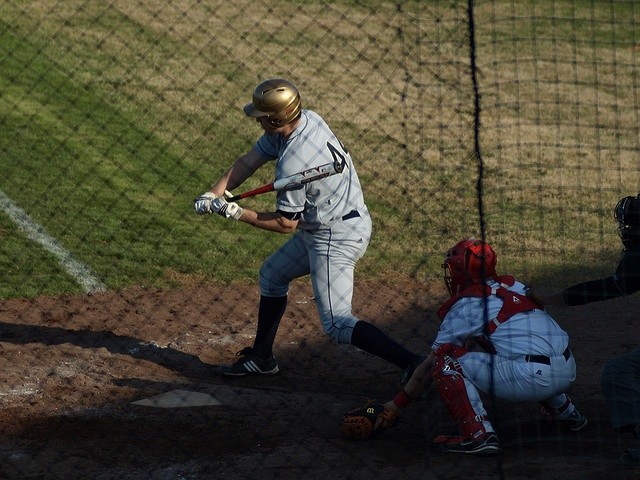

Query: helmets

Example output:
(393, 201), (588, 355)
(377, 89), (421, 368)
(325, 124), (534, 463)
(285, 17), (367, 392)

(441, 238), (496, 296)
(613, 195), (640, 250)
(242, 77), (302, 132)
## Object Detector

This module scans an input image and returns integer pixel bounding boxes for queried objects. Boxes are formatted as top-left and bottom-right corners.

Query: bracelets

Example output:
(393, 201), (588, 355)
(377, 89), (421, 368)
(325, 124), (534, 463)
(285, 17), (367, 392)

(392, 387), (413, 410)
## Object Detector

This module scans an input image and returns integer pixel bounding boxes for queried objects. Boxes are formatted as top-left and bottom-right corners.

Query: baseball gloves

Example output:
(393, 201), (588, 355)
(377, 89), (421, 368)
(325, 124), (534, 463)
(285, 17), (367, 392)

(340, 399), (396, 438)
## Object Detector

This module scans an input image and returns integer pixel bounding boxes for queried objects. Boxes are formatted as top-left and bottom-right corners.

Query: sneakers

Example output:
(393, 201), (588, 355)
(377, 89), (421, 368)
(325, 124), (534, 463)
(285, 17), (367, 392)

(222, 346), (280, 376)
(446, 431), (501, 455)
(401, 354), (429, 385)
(616, 448), (640, 464)
(542, 400), (589, 433)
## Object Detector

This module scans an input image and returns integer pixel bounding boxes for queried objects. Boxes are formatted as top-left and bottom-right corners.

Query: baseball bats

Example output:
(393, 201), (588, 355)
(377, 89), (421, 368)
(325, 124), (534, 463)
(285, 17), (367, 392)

(226, 161), (342, 202)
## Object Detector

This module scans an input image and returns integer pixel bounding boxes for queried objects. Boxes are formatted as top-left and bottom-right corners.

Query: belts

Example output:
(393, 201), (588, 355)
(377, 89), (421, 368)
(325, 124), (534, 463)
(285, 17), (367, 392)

(526, 348), (572, 366)
(340, 210), (360, 221)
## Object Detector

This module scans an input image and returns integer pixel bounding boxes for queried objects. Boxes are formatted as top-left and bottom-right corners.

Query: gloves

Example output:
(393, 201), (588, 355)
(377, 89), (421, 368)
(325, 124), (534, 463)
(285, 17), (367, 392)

(213, 188), (242, 221)
(193, 192), (215, 215)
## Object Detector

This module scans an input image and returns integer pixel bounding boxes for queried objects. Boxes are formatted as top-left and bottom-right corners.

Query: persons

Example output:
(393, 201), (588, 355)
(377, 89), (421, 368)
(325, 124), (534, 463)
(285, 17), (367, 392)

(373, 240), (589, 455)
(600, 347), (640, 467)
(546, 193), (640, 307)
(193, 79), (429, 384)
